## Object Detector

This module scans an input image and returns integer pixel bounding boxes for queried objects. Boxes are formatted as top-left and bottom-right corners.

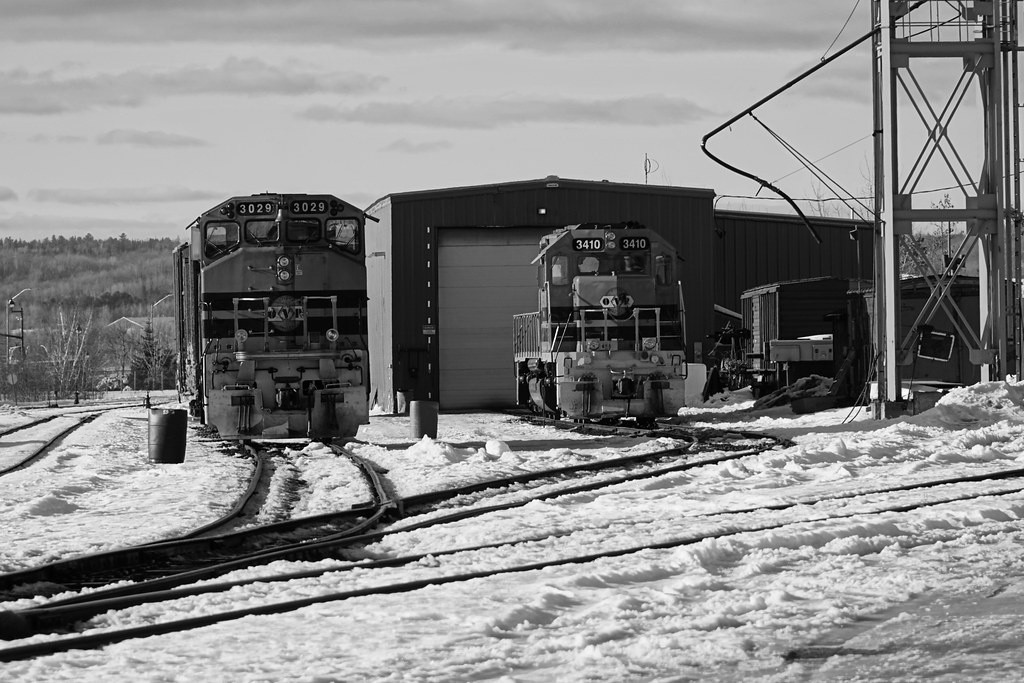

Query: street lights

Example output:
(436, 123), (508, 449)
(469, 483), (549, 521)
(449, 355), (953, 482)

(151, 293), (175, 390)
(9, 299), (24, 359)
(73, 323), (82, 404)
(5, 288), (31, 368)
(85, 351), (90, 399)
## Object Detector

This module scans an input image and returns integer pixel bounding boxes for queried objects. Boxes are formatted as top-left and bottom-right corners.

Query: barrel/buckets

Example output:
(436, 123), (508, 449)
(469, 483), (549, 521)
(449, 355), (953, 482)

(409, 400), (439, 441)
(148, 407), (187, 464)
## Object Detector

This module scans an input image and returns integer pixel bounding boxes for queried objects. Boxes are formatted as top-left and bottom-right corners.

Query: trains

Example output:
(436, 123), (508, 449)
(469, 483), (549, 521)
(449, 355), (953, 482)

(512, 222), (688, 430)
(172, 191), (371, 446)
